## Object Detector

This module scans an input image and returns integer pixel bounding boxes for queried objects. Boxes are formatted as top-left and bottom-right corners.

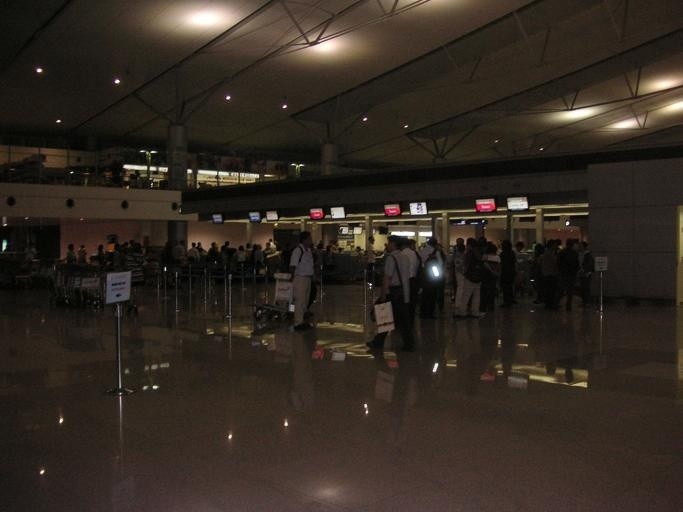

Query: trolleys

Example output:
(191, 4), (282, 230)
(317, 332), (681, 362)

(250, 280), (321, 322)
(52, 262), (106, 311)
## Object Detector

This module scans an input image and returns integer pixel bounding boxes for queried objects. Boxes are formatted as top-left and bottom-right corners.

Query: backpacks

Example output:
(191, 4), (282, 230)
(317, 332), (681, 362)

(423, 248), (444, 288)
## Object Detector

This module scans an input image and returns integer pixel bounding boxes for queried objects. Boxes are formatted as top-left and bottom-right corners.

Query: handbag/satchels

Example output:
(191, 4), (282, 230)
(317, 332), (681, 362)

(374, 284), (405, 334)
(307, 280), (317, 307)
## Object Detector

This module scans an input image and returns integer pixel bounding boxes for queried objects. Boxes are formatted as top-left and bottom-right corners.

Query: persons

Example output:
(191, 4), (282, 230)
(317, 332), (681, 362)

(21, 231), (592, 351)
